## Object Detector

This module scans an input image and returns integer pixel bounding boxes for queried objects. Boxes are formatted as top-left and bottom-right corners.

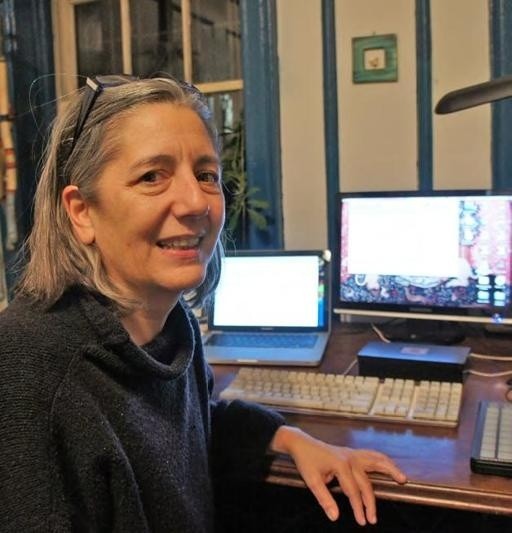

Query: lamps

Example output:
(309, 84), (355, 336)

(435, 75), (512, 114)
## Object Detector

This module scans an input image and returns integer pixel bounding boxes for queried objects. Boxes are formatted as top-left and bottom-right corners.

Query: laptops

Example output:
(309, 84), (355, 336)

(197, 250), (332, 367)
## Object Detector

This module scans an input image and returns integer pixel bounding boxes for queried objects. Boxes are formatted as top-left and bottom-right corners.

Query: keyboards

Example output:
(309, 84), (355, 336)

(218, 368), (463, 430)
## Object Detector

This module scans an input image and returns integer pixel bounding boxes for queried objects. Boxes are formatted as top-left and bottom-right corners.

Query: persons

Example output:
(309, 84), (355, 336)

(1, 69), (410, 532)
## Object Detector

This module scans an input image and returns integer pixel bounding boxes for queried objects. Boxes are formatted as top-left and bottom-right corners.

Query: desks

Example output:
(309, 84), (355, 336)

(209, 320), (512, 518)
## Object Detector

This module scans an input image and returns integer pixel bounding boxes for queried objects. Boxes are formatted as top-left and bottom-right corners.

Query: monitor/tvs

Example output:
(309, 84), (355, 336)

(333, 189), (512, 346)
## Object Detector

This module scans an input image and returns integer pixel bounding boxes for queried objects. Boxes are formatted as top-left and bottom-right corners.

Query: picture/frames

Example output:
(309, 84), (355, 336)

(351, 33), (399, 84)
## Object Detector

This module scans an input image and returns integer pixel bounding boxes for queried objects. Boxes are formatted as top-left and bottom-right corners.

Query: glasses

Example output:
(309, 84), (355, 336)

(67, 69), (205, 159)
